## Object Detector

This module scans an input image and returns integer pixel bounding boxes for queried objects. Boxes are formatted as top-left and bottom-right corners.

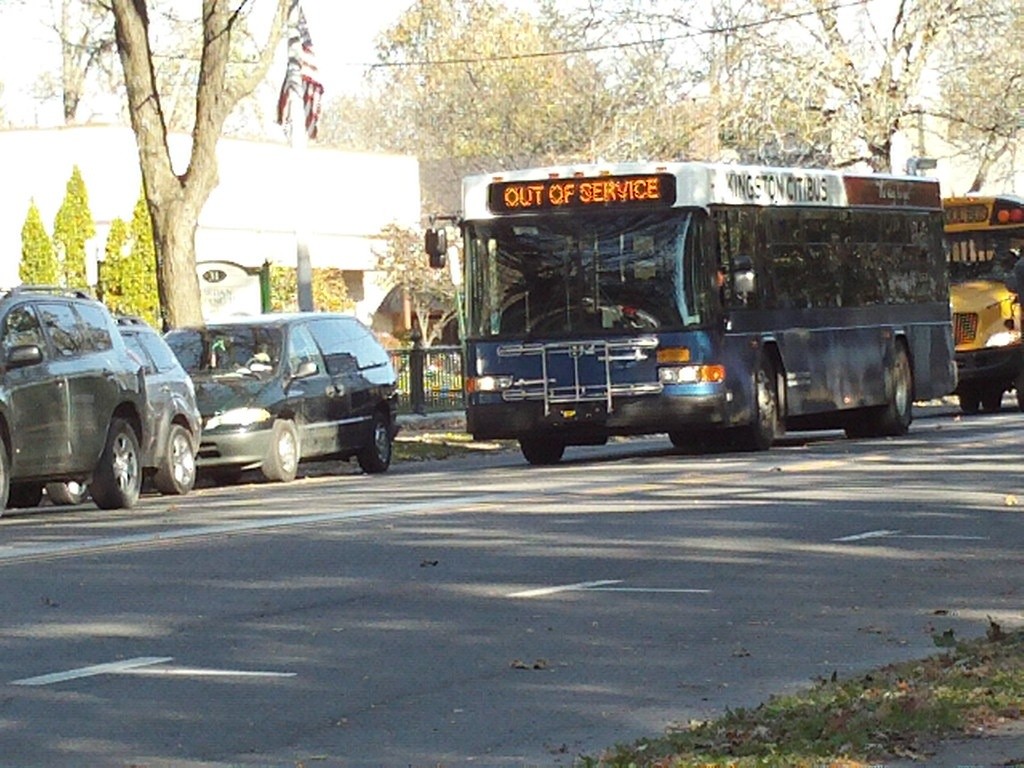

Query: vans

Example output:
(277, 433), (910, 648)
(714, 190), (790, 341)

(156, 312), (405, 484)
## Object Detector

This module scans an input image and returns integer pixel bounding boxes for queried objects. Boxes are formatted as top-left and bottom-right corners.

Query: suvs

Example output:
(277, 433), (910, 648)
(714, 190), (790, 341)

(1, 281), (149, 510)
(41, 313), (204, 508)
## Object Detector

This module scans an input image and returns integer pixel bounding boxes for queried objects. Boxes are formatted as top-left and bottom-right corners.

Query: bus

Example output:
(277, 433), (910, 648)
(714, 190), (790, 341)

(424, 159), (961, 465)
(926, 191), (1024, 414)
